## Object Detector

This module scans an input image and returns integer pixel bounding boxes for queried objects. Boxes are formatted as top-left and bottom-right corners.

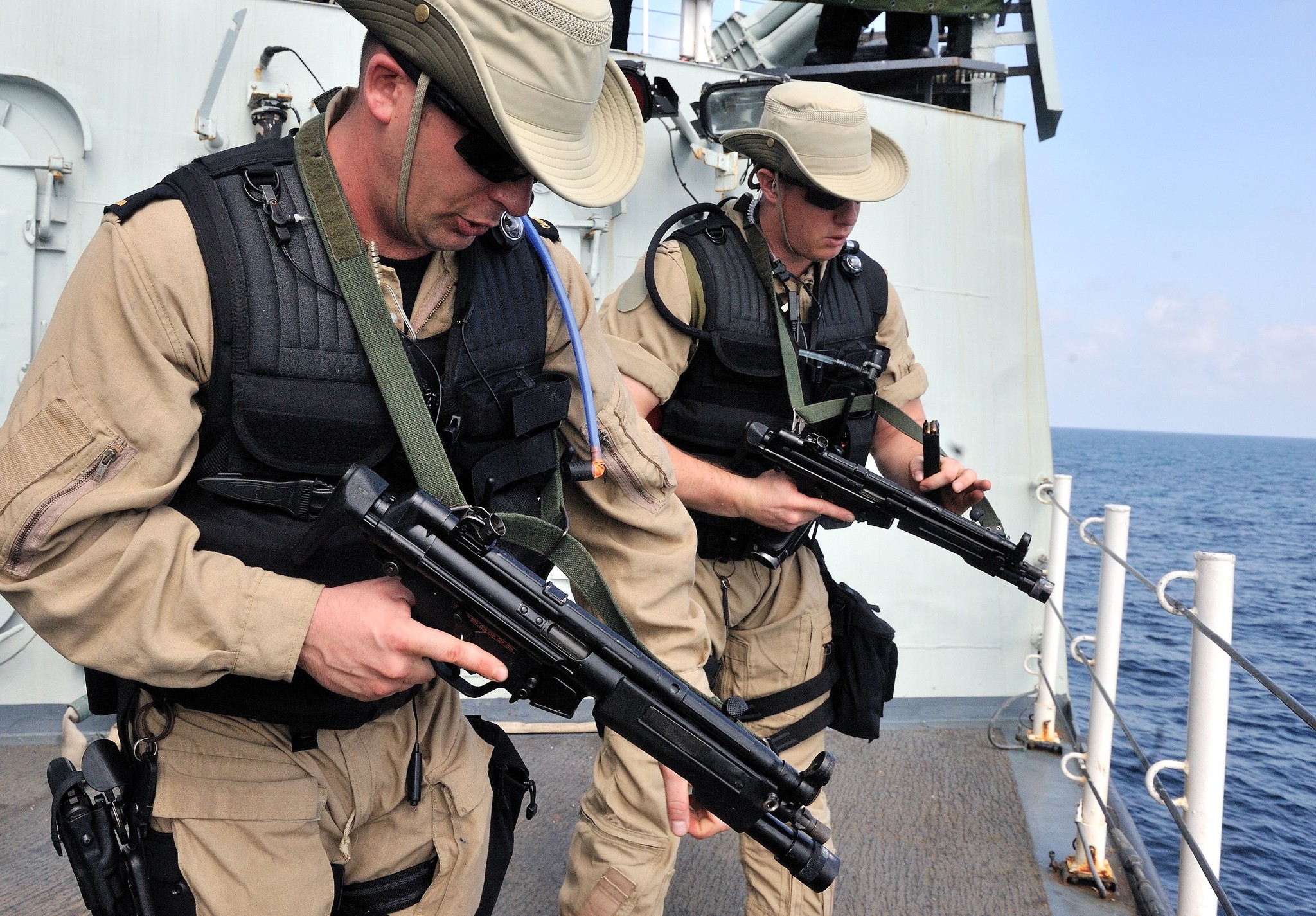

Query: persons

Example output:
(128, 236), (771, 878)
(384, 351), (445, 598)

(0, 0), (743, 915)
(553, 73), (995, 914)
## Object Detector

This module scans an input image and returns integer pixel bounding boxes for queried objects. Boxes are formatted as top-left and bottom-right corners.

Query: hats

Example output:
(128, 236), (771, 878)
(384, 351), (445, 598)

(719, 81), (910, 203)
(336, 0), (646, 209)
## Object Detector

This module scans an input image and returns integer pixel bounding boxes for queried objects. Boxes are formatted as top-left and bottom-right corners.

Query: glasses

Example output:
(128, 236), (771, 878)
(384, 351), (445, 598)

(382, 43), (539, 184)
(764, 165), (861, 210)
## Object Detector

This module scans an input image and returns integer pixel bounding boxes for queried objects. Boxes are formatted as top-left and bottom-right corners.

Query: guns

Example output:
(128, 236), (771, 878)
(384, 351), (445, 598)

(742, 415), (1056, 605)
(42, 752), (201, 915)
(337, 454), (839, 897)
